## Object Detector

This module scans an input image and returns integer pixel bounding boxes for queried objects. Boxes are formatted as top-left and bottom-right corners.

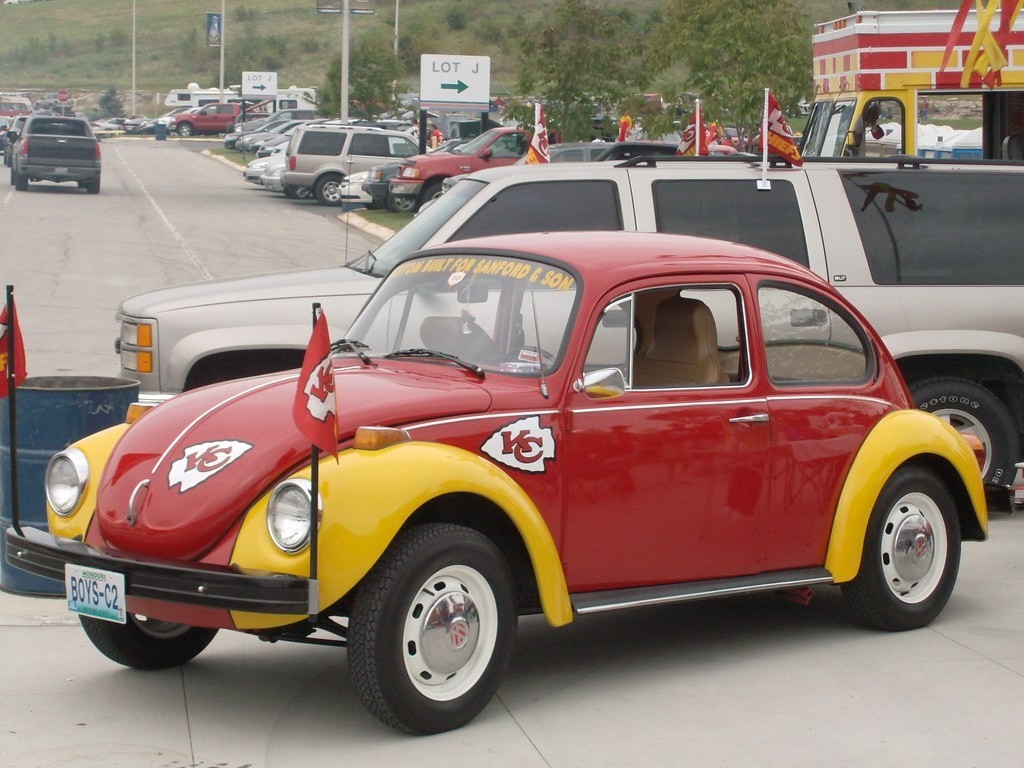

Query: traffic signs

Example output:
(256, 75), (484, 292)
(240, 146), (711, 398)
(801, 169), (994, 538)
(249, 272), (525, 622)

(420, 55), (491, 113)
(242, 71), (278, 101)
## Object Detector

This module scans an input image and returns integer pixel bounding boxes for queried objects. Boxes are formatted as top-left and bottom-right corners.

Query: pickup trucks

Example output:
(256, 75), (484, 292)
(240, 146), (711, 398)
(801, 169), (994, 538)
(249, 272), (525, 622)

(167, 102), (268, 137)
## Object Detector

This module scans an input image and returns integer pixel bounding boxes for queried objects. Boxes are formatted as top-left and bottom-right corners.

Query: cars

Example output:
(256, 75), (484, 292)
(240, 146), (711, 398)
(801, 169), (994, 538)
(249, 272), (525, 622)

(91, 105), (216, 138)
(4, 231), (990, 739)
(224, 92), (803, 223)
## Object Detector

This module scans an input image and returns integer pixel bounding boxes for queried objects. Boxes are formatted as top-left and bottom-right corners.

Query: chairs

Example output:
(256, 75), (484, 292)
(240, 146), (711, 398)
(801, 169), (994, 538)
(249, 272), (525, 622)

(623, 297), (730, 390)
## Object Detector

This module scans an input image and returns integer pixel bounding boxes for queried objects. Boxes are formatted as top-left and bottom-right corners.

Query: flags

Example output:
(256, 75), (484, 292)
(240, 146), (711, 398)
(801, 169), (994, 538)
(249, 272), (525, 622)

(294, 311), (339, 467)
(0, 295), (27, 397)
(526, 86), (805, 167)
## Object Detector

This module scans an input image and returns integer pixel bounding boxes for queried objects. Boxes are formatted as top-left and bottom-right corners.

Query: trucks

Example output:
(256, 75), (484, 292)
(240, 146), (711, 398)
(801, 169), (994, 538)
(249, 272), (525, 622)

(792, 8), (1024, 173)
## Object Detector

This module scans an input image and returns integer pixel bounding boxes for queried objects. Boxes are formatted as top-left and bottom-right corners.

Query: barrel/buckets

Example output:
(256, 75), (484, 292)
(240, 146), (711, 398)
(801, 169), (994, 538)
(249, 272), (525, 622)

(155, 124), (166, 141)
(0, 377), (140, 599)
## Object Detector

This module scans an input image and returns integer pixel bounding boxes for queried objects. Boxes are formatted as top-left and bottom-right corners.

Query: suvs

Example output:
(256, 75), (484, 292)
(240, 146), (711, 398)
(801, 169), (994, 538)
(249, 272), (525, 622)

(113, 151), (1024, 509)
(0, 101), (36, 168)
(6, 115), (102, 195)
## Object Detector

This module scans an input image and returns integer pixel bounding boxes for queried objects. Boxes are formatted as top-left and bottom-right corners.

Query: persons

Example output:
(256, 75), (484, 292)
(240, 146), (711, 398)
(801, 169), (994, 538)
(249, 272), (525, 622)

(428, 123), (444, 149)
(495, 95), (503, 106)
(922, 101), (929, 120)
(410, 119), (419, 137)
